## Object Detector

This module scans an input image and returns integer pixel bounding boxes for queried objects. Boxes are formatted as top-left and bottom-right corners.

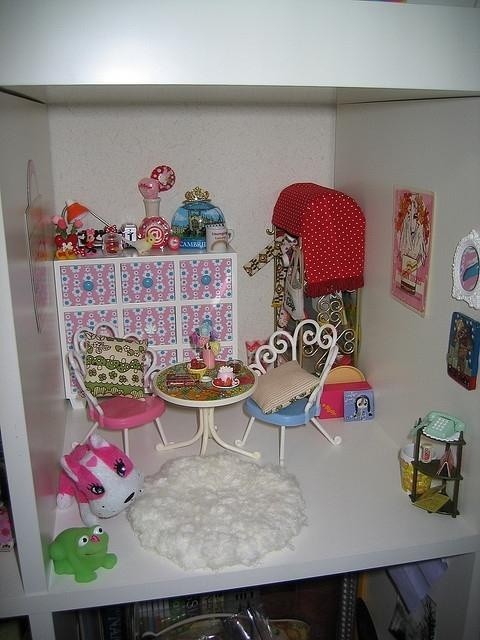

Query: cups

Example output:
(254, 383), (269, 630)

(399, 443), (437, 495)
(206, 223), (233, 253)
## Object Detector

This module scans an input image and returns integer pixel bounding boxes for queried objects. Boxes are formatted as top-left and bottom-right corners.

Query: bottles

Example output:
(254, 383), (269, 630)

(102, 232), (124, 257)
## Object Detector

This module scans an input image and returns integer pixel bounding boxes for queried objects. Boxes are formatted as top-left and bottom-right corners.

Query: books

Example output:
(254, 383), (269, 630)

(71, 585), (259, 640)
